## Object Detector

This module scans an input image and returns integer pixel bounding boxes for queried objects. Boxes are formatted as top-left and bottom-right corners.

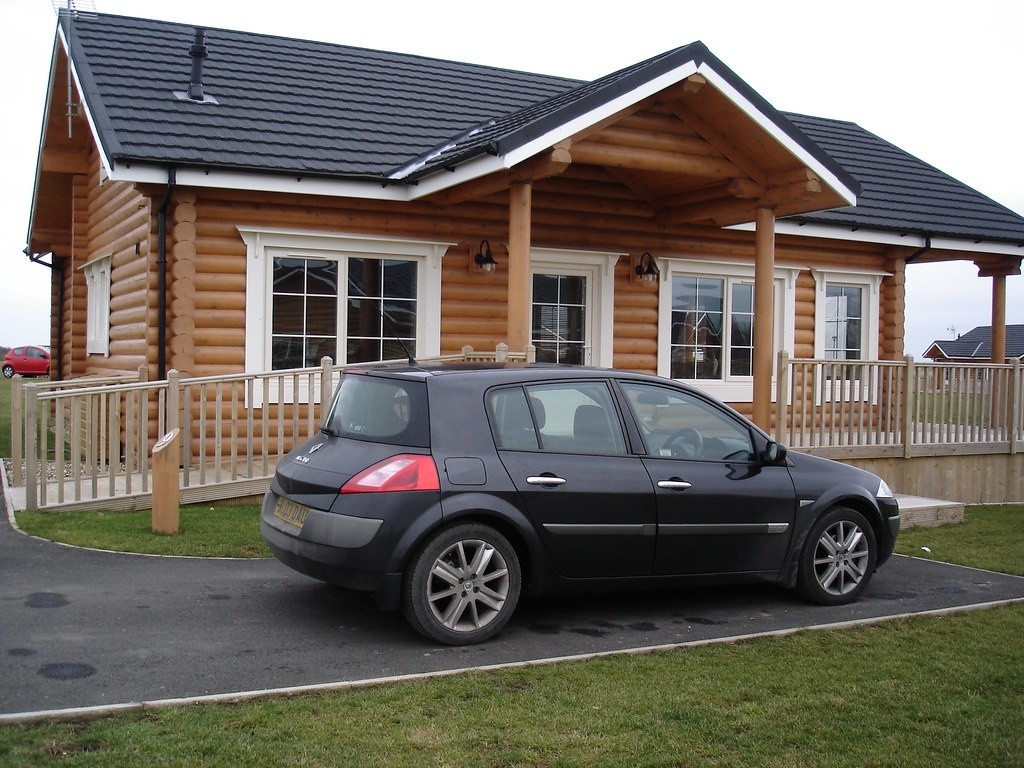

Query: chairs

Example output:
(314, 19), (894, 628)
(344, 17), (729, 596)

(509, 397), (614, 454)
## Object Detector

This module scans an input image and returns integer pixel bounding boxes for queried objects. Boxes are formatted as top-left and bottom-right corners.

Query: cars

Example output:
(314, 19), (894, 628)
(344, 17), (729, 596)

(257, 358), (902, 644)
(1, 345), (51, 378)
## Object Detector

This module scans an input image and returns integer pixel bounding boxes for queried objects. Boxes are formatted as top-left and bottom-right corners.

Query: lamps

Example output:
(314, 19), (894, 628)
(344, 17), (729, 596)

(636, 252), (660, 285)
(475, 240), (498, 275)
